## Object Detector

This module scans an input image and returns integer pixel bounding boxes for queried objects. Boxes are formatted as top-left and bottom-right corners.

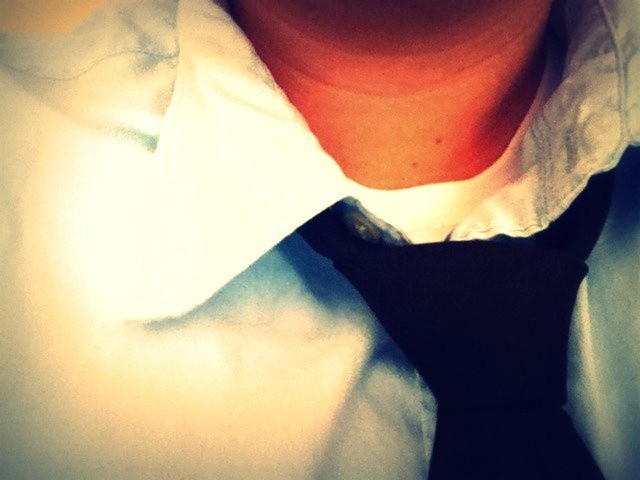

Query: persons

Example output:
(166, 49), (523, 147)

(0, 1), (638, 480)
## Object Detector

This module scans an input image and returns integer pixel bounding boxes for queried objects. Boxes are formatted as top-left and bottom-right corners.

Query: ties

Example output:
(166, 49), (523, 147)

(298, 171), (617, 478)
